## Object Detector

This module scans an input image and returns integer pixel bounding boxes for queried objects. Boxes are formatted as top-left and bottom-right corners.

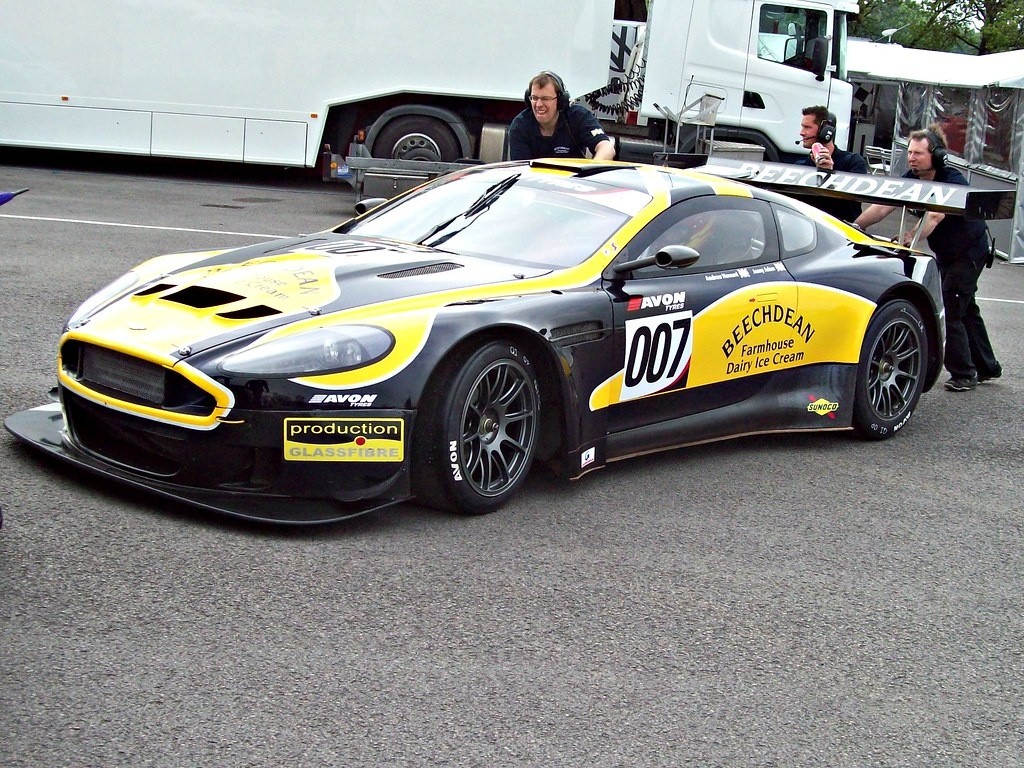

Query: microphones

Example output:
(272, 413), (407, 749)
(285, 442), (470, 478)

(796, 136), (816, 145)
(913, 168), (939, 174)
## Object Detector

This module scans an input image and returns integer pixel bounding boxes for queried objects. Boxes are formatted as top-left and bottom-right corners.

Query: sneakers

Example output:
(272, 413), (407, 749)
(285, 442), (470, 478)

(977, 361), (1003, 384)
(944, 370), (978, 391)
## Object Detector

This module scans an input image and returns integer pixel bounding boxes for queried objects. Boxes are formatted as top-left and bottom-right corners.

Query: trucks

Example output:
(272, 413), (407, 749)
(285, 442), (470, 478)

(0, 0), (861, 192)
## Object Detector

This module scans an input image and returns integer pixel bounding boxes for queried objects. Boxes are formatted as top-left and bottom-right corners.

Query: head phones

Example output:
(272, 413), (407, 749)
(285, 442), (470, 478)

(919, 128), (948, 169)
(816, 112), (837, 144)
(525, 71), (570, 111)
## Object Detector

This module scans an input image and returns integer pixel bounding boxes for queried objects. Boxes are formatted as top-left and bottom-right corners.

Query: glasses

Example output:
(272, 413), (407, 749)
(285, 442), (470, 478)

(528, 96), (558, 103)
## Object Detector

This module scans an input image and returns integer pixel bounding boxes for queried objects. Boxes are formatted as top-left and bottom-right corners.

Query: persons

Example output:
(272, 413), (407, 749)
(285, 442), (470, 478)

(509, 70), (616, 160)
(844, 121), (1002, 391)
(775, 106), (866, 232)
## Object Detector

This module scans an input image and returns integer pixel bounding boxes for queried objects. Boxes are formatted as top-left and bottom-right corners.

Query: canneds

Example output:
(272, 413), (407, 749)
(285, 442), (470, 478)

(812, 143), (826, 164)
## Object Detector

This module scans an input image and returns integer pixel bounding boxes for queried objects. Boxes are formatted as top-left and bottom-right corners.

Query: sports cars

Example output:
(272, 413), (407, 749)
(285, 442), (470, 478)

(1, 158), (972, 532)
